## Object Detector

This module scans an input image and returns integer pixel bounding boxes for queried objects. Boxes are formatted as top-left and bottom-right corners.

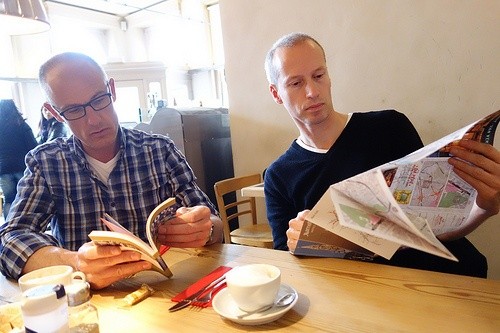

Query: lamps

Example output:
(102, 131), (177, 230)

(0, 0), (51, 35)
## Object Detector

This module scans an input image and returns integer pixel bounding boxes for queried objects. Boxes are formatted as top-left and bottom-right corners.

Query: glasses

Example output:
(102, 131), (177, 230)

(52, 81), (111, 121)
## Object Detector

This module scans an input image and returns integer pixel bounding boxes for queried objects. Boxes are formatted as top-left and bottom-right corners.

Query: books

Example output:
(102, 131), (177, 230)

(88, 197), (177, 279)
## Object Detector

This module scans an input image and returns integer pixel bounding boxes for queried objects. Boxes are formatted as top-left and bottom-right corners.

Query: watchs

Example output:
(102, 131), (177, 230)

(211, 217), (217, 243)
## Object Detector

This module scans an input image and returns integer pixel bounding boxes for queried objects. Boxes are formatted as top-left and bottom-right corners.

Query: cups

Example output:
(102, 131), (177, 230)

(19, 265), (86, 293)
(226, 264), (280, 313)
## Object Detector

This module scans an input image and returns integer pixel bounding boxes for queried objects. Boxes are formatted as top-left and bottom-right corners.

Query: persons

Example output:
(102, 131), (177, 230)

(0, 100), (38, 221)
(0, 52), (224, 290)
(264, 32), (500, 280)
(36, 103), (67, 144)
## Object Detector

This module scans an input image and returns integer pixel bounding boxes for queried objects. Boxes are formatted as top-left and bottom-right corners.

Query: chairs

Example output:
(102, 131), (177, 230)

(213, 173), (274, 248)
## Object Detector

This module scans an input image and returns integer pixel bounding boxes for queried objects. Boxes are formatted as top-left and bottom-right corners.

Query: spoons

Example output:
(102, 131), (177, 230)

(238, 293), (295, 319)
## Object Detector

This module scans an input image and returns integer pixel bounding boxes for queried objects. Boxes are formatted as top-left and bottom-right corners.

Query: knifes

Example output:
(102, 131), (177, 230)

(169, 273), (226, 311)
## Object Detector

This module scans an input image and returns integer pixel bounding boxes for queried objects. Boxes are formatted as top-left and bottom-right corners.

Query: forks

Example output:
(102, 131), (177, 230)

(189, 279), (226, 312)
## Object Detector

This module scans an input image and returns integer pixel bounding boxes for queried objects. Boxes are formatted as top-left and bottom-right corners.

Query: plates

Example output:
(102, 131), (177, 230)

(212, 284), (298, 325)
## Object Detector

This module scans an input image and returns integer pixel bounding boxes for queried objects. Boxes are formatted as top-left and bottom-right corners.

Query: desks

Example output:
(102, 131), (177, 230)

(0, 244), (500, 333)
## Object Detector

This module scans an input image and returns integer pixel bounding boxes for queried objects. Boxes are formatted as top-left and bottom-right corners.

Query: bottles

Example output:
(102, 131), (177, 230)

(67, 282), (99, 333)
